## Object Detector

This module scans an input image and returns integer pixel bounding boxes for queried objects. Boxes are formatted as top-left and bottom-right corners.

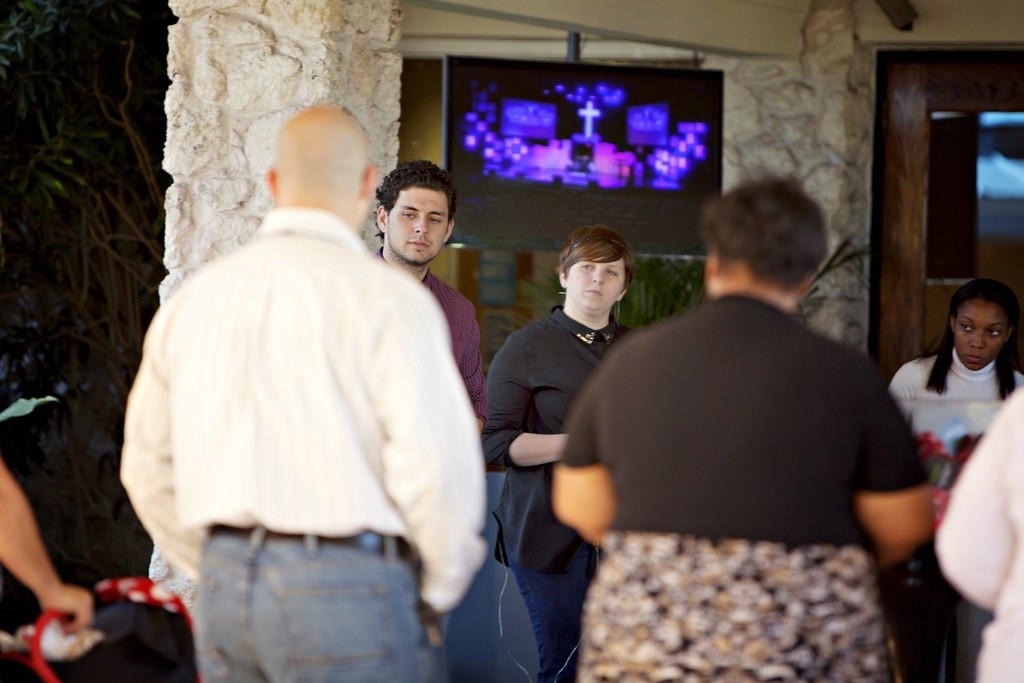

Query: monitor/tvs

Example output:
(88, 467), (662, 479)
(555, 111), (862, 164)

(441, 54), (724, 259)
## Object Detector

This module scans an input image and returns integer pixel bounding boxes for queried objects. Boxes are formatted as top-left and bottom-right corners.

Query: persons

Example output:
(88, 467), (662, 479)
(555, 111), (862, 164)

(120, 107), (486, 683)
(370, 159), (488, 439)
(478, 224), (637, 683)
(886, 278), (1024, 402)
(0, 456), (94, 635)
(934, 384), (1024, 683)
(551, 176), (933, 683)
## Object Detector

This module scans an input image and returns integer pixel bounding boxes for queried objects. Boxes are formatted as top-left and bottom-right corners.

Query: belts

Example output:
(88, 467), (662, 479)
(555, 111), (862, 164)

(210, 522), (413, 560)
